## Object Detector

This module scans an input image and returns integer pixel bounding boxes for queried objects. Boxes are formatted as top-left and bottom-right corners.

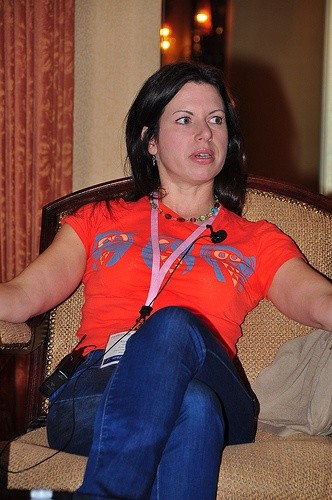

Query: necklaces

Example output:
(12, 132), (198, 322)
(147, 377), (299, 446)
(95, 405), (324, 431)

(149, 192), (220, 222)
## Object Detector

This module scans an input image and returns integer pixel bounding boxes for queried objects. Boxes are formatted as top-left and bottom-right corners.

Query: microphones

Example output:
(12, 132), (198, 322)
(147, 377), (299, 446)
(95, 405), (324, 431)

(211, 230), (227, 243)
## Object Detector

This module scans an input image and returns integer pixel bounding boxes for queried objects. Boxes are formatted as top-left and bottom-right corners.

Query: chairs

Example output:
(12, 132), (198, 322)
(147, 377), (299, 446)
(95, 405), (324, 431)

(0, 172), (332, 500)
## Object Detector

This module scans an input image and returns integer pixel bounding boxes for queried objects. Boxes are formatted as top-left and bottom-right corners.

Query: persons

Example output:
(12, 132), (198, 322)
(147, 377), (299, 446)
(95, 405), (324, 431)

(0, 64), (332, 500)
